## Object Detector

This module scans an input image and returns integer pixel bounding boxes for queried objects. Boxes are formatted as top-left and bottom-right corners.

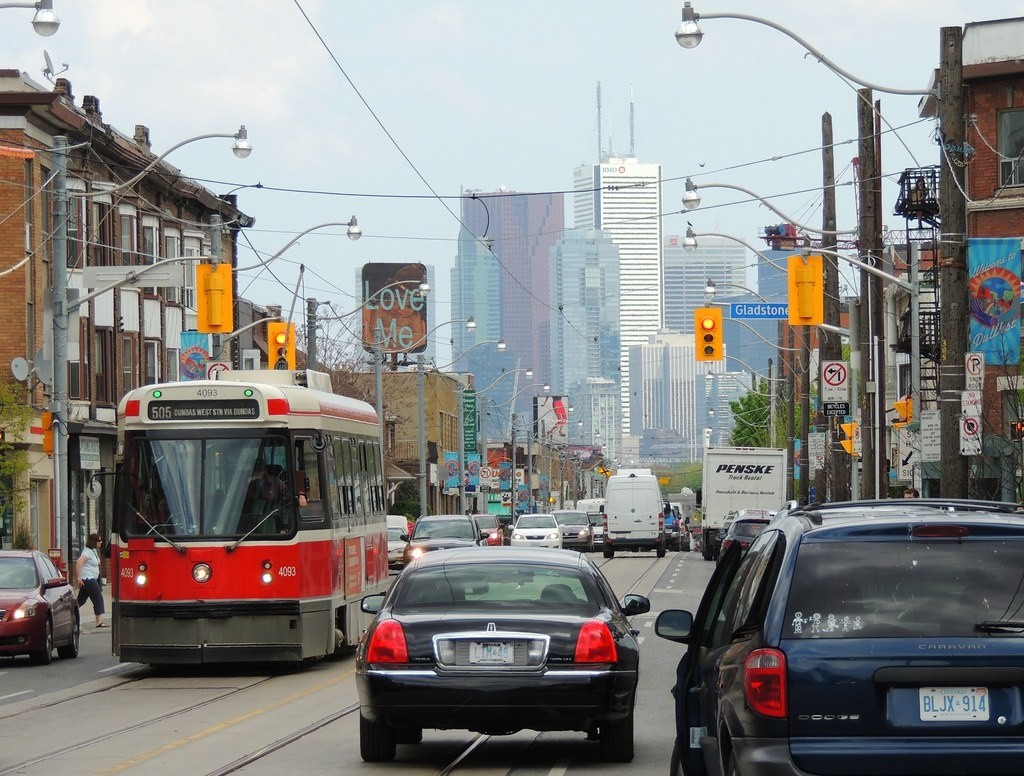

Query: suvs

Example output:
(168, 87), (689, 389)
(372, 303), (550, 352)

(654, 498), (1024, 776)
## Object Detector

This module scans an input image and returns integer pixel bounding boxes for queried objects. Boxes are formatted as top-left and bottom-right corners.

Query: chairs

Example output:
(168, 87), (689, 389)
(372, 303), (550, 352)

(539, 585), (579, 602)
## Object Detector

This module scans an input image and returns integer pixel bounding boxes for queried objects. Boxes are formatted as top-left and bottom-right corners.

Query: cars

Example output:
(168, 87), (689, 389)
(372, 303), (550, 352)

(665, 503), (694, 552)
(384, 510), (606, 566)
(0, 550), (81, 665)
(357, 545), (651, 764)
(716, 513), (772, 561)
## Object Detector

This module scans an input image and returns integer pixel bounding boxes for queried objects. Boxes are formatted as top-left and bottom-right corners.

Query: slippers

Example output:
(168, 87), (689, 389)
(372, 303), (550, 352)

(96, 622), (110, 627)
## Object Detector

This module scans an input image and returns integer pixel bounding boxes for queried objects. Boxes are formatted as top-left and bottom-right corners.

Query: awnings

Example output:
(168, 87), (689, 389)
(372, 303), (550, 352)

(387, 462), (416, 499)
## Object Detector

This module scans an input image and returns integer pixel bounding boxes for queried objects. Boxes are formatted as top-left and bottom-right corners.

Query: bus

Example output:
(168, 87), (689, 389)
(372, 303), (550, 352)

(85, 264), (391, 665)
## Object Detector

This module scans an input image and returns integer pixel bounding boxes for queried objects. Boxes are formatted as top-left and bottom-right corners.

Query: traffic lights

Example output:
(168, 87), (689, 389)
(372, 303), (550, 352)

(266, 321), (295, 371)
(694, 309), (725, 363)
(892, 399), (911, 428)
(840, 423), (856, 456)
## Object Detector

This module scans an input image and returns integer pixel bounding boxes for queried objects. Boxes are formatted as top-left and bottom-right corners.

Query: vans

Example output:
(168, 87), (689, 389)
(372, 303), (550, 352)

(604, 468), (666, 560)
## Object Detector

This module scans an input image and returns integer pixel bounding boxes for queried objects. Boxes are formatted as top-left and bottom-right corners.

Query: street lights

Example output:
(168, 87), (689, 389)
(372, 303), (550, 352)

(676, 0), (942, 100)
(208, 215), (363, 375)
(679, 176), (914, 400)
(302, 279), (607, 516)
(45, 125), (252, 576)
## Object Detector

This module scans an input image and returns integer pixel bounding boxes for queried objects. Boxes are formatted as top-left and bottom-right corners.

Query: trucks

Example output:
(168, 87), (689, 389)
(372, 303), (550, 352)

(701, 444), (786, 561)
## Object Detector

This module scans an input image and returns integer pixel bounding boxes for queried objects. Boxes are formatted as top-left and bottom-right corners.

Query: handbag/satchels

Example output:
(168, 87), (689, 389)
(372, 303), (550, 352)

(97, 574), (103, 591)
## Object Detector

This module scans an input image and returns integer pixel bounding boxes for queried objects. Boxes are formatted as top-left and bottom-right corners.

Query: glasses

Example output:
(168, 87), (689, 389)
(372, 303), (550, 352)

(97, 540), (102, 542)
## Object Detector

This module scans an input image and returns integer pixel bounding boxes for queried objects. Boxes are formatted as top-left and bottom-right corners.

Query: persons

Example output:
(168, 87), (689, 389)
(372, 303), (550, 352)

(541, 396), (568, 440)
(904, 488), (919, 500)
(248, 465), (308, 508)
(74, 533), (110, 629)
